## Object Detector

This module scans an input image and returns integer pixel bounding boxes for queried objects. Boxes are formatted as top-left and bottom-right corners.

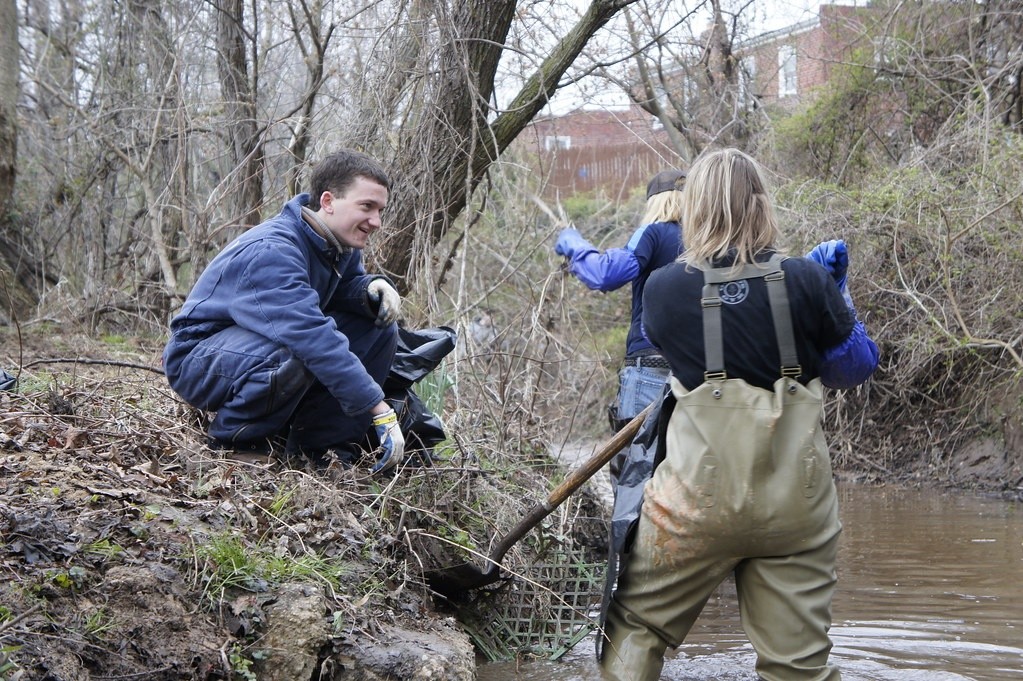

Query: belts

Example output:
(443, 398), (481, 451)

(624, 357), (672, 369)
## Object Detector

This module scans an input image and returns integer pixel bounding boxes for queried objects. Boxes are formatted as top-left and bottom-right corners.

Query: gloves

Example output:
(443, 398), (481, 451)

(368, 278), (401, 328)
(556, 228), (640, 293)
(804, 239), (880, 390)
(371, 409), (404, 477)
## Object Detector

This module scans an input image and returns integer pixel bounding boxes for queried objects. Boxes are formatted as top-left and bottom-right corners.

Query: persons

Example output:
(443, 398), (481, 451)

(556, 171), (685, 495)
(606, 149), (854, 681)
(162, 150), (406, 475)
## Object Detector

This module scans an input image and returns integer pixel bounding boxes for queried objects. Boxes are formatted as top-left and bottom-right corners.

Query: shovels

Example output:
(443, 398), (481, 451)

(420, 398), (656, 592)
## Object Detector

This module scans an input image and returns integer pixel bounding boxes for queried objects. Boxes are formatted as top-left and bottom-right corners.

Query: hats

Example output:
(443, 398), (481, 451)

(646, 171), (688, 200)
